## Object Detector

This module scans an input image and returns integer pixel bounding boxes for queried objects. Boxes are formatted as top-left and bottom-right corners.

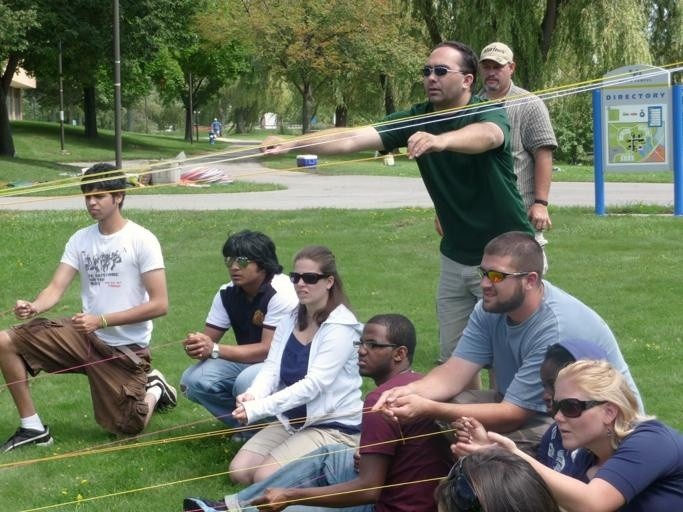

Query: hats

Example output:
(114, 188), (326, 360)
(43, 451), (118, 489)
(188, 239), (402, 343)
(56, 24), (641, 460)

(481, 41), (515, 67)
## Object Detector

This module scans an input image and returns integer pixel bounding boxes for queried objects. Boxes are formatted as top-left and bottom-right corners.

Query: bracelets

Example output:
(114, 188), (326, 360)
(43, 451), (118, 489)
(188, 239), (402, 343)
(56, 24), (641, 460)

(99, 314), (108, 328)
(534, 198), (548, 206)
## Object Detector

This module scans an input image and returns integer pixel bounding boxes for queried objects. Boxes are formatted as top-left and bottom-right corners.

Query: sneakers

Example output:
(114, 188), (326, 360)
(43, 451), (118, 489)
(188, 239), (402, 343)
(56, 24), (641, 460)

(182, 496), (224, 511)
(2, 424), (53, 454)
(146, 369), (179, 409)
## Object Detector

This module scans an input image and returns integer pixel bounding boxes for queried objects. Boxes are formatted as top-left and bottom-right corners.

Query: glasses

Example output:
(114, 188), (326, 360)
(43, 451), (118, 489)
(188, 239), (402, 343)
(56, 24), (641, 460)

(422, 66), (463, 76)
(477, 267), (528, 284)
(446, 457), (479, 509)
(224, 257), (256, 267)
(551, 399), (610, 418)
(289, 271), (326, 285)
(353, 340), (398, 350)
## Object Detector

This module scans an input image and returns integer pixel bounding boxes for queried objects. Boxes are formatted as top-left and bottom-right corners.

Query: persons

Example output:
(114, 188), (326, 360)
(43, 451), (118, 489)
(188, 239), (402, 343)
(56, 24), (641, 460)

(180, 229), (306, 443)
(453, 332), (611, 487)
(471, 41), (557, 281)
(451, 358), (683, 511)
(260, 41), (549, 393)
(184, 315), (455, 512)
(228, 246), (364, 485)
(211, 118), (222, 137)
(0, 164), (178, 456)
(371, 230), (645, 449)
(434, 447), (561, 512)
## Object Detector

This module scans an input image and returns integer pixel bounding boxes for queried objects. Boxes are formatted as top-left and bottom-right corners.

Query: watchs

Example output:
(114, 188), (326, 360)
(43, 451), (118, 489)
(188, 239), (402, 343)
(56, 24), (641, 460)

(211, 343), (219, 359)
